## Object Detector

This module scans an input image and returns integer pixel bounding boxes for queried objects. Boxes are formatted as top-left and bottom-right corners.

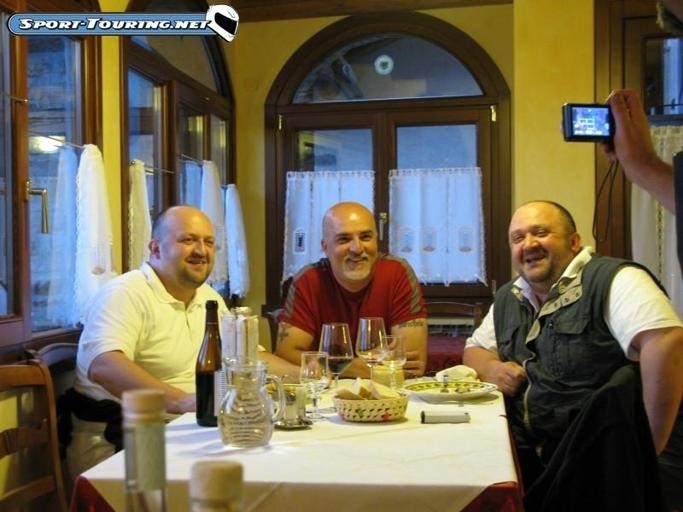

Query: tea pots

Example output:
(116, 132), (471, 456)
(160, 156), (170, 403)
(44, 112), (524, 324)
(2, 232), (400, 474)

(217, 356), (287, 450)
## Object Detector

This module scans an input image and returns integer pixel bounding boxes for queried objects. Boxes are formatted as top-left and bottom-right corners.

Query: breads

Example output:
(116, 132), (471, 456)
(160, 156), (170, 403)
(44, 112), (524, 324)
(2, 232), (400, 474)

(337, 377), (401, 400)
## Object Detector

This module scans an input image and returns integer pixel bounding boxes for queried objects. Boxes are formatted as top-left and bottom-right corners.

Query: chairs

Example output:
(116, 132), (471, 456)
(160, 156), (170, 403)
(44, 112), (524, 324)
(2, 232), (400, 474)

(0, 362), (67, 512)
(427, 301), (482, 374)
(24, 340), (78, 480)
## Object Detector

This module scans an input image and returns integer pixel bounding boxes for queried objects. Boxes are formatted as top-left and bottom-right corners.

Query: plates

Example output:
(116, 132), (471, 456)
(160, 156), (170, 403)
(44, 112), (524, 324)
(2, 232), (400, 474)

(404, 380), (499, 403)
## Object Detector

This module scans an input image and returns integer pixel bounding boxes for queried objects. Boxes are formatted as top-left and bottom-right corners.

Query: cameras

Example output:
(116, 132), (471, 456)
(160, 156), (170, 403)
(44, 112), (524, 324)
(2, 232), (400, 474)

(562, 102), (617, 142)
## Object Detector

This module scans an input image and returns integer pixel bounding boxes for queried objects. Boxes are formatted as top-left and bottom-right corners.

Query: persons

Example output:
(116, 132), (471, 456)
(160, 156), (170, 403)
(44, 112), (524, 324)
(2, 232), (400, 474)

(66, 205), (301, 483)
(601, 89), (676, 216)
(463, 200), (683, 512)
(276, 202), (428, 379)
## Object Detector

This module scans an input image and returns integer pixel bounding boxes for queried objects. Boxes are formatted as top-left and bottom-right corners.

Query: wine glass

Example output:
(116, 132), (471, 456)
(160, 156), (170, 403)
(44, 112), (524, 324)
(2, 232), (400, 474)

(299, 316), (406, 422)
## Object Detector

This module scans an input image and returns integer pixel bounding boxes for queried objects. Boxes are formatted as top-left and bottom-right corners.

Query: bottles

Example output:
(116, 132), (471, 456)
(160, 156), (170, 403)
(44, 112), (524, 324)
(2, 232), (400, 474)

(195, 299), (222, 427)
(121, 387), (244, 511)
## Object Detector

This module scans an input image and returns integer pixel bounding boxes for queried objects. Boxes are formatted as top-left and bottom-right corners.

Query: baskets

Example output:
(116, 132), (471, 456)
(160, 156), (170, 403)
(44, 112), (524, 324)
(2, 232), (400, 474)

(332, 394), (408, 421)
